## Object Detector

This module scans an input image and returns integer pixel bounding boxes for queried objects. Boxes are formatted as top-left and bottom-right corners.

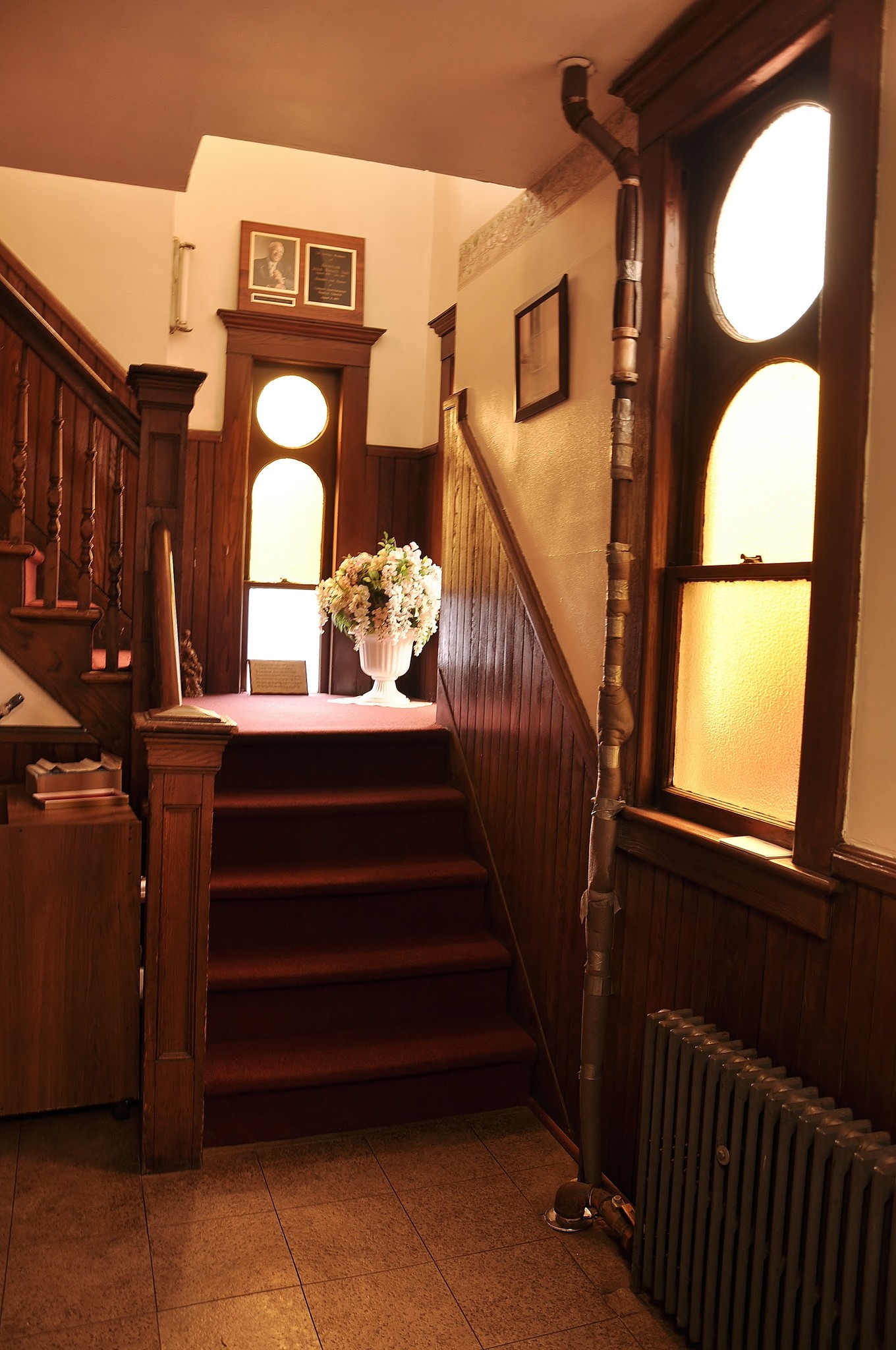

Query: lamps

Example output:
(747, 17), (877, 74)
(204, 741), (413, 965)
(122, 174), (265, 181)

(170, 235), (195, 336)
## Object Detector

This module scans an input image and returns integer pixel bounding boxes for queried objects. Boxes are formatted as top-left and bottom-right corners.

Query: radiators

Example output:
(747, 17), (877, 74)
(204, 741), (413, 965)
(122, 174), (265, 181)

(627, 1001), (896, 1350)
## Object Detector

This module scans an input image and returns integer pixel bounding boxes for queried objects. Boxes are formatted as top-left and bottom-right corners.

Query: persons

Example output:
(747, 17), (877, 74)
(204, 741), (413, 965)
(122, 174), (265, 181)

(253, 241), (287, 289)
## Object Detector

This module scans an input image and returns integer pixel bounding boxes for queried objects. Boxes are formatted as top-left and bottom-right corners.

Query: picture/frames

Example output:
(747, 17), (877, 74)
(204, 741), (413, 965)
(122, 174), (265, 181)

(303, 241), (357, 311)
(512, 272), (571, 424)
(247, 229), (301, 295)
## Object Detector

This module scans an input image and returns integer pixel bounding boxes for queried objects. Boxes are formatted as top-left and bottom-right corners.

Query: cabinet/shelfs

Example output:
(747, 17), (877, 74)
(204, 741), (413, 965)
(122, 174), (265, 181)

(1, 782), (142, 1120)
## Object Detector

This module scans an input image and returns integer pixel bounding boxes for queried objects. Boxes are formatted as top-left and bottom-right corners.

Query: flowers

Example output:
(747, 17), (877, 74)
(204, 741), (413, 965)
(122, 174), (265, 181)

(314, 530), (445, 657)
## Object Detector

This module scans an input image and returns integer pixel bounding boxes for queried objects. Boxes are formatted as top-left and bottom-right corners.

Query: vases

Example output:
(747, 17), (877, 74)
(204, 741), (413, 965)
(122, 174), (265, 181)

(354, 625), (412, 705)
